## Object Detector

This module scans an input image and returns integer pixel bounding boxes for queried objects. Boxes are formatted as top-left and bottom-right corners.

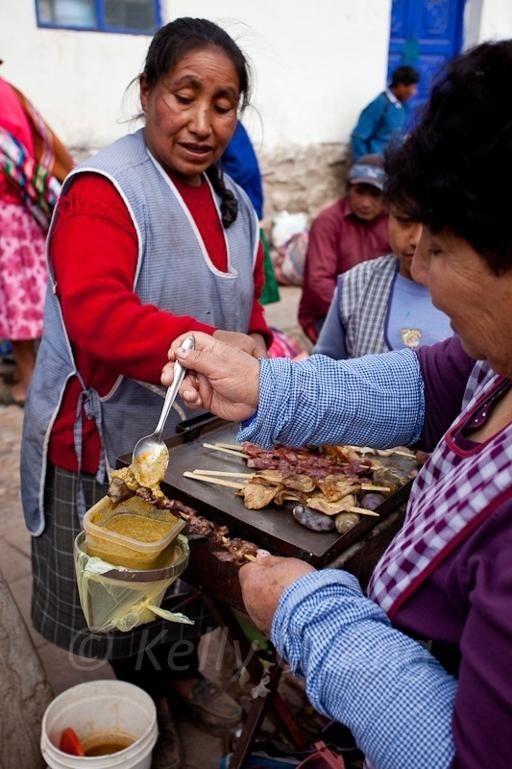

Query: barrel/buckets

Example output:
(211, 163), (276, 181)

(41, 678), (157, 768)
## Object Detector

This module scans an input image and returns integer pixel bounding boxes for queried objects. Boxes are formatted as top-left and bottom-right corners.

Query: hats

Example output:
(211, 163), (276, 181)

(348, 165), (386, 192)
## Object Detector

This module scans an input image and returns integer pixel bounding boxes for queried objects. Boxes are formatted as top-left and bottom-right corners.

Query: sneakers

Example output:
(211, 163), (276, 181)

(152, 709), (185, 769)
(182, 674), (244, 736)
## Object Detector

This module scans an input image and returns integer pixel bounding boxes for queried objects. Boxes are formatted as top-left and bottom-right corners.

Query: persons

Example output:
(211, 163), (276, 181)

(218, 119), (283, 306)
(346, 65), (424, 160)
(0, 56), (75, 408)
(18, 15), (274, 769)
(308, 183), (457, 362)
(160, 39), (512, 769)
(297, 162), (390, 345)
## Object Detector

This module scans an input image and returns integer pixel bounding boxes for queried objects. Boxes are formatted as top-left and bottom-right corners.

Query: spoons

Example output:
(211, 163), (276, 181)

(131, 335), (196, 488)
